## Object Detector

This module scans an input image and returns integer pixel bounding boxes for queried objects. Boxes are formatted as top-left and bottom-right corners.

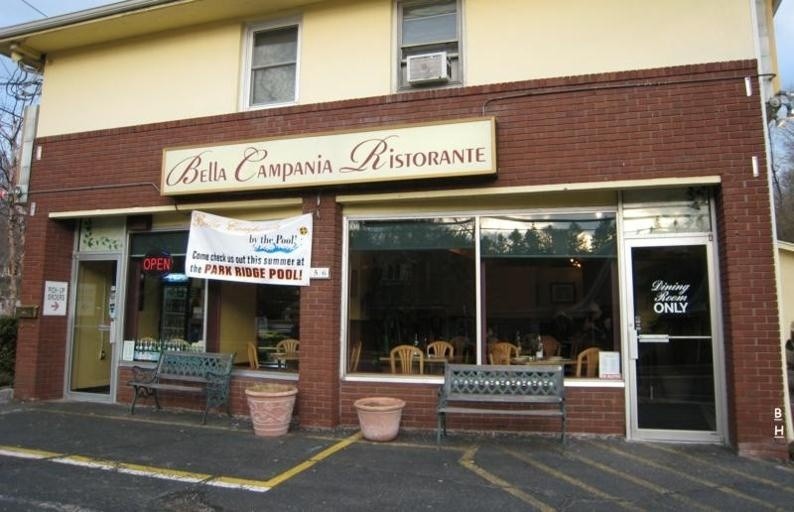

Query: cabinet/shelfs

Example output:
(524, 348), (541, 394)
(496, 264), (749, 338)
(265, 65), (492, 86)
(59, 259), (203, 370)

(159, 272), (190, 342)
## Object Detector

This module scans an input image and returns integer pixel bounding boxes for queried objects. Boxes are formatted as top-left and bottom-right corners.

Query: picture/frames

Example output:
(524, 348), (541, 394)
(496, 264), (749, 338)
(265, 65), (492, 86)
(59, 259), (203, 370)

(550, 282), (576, 305)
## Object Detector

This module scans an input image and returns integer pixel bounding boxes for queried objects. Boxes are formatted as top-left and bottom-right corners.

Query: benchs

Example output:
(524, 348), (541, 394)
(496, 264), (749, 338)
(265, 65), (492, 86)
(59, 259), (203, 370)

(127, 349), (238, 425)
(436, 363), (566, 447)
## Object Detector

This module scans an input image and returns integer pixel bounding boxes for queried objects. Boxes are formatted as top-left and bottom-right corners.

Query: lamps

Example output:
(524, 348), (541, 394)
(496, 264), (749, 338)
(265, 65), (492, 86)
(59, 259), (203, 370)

(570, 258), (585, 273)
(764, 91), (794, 128)
(127, 214), (152, 231)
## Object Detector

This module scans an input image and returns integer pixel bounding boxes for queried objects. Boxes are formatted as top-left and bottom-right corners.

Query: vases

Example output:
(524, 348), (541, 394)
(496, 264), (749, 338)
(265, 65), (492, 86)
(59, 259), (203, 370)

(244, 383), (298, 438)
(353, 397), (406, 441)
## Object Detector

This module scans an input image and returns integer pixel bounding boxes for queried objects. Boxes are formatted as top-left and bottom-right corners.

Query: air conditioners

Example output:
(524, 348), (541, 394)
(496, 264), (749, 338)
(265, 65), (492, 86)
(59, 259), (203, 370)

(407, 50), (452, 84)
